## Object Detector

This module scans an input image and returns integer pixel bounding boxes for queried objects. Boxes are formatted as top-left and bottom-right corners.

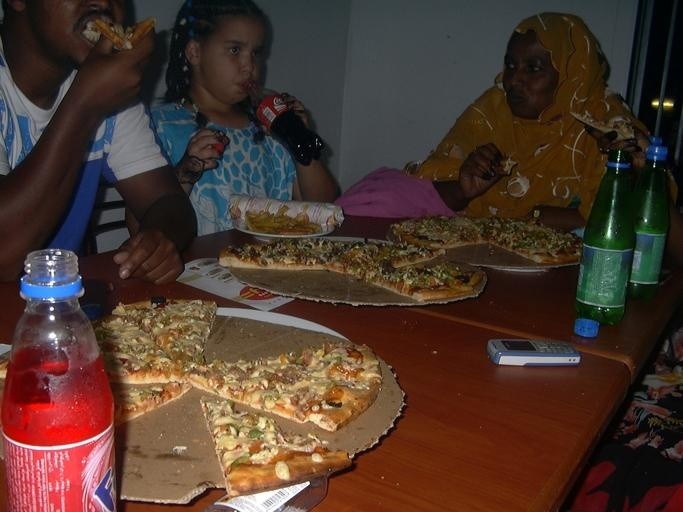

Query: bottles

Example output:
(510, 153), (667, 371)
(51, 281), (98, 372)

(574, 145), (634, 326)
(245, 78), (326, 166)
(0, 247), (118, 511)
(628, 136), (668, 302)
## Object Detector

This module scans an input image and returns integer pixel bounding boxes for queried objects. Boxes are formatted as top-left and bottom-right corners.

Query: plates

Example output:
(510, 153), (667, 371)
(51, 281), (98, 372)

(233, 212), (340, 241)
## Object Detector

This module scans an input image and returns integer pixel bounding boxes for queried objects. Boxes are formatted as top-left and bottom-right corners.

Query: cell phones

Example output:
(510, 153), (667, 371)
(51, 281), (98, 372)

(487, 339), (582, 367)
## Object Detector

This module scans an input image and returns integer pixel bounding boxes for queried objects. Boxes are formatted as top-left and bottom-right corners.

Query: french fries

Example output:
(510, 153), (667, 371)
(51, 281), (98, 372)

(245, 210), (322, 236)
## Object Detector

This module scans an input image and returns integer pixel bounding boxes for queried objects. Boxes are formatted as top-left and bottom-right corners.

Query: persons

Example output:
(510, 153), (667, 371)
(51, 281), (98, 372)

(1, 0), (197, 286)
(407, 11), (682, 260)
(124, 1), (343, 241)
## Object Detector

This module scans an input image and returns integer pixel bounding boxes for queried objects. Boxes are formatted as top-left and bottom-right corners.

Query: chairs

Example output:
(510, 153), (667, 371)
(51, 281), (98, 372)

(83, 178), (131, 257)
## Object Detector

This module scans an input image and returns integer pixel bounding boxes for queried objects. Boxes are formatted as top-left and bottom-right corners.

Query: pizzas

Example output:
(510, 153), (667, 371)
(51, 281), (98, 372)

(390, 214), (585, 265)
(218, 241), (484, 302)
(0, 297), (383, 497)
(83, 19), (157, 52)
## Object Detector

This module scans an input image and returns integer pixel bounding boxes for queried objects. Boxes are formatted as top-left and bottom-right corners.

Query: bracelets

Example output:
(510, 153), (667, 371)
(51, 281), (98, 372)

(532, 204), (544, 225)
